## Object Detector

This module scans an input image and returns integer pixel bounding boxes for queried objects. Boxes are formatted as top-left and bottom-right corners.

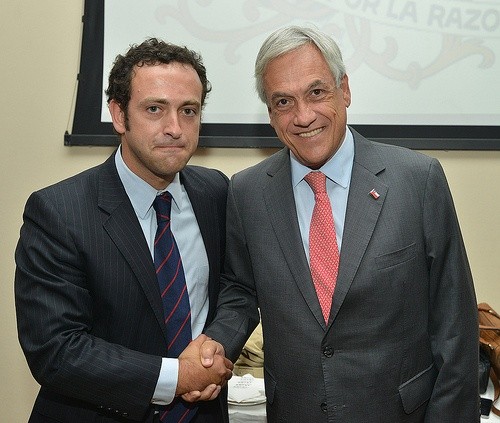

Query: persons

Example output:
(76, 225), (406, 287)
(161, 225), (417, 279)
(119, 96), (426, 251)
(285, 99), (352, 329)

(173, 22), (483, 422)
(13, 35), (233, 423)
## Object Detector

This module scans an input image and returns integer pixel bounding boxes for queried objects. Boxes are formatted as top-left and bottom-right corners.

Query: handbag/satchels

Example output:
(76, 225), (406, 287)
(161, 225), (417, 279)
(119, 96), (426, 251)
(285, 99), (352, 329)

(475, 303), (500, 416)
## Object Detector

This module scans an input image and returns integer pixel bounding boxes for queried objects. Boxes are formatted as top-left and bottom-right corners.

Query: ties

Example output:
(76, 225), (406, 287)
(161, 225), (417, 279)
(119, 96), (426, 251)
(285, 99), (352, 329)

(148, 192), (201, 423)
(303, 172), (340, 323)
